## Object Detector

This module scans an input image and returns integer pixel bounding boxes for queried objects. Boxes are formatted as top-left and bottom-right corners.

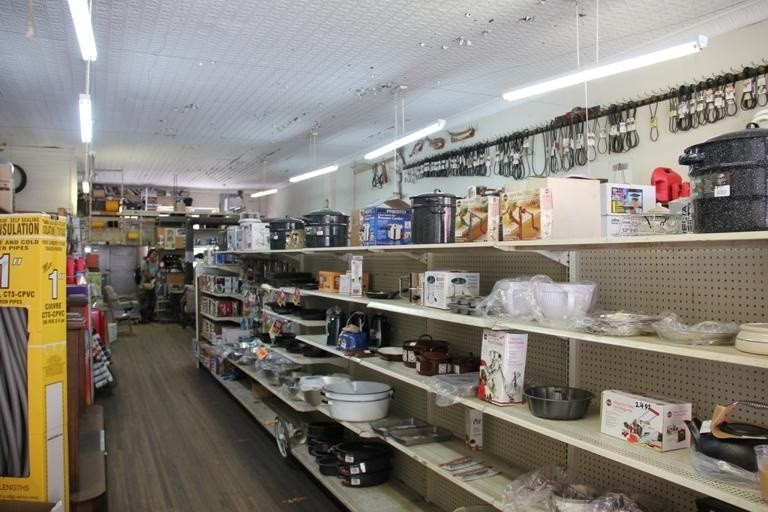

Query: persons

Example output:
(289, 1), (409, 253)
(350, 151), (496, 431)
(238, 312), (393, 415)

(139, 249), (159, 324)
(183, 254), (203, 284)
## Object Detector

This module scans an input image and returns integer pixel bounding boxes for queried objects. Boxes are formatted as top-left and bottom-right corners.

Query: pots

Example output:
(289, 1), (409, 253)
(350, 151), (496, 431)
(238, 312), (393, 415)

(388, 223), (402, 240)
(452, 352), (479, 374)
(265, 216), (305, 249)
(417, 348), (447, 374)
(303, 210), (350, 247)
(403, 340), (450, 368)
(679, 122), (768, 232)
(410, 189), (456, 243)
(300, 375), (395, 423)
(365, 289), (407, 298)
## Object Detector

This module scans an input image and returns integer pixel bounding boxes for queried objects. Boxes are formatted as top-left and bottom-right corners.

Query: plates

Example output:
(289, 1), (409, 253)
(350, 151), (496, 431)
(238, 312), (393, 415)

(586, 314), (658, 337)
(377, 346), (404, 360)
(655, 330), (735, 346)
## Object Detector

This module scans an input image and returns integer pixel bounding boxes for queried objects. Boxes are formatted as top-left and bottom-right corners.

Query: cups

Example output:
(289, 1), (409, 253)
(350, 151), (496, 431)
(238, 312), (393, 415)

(534, 282), (596, 328)
(499, 280), (535, 317)
(755, 445), (768, 503)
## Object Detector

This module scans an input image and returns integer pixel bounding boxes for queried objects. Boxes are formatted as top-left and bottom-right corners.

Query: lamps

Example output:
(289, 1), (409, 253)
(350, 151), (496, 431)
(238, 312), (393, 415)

(499, 35), (708, 102)
(250, 188), (279, 198)
(81, 180), (90, 194)
(288, 162), (338, 183)
(67, 0), (98, 61)
(79, 94), (94, 144)
(361, 118), (446, 161)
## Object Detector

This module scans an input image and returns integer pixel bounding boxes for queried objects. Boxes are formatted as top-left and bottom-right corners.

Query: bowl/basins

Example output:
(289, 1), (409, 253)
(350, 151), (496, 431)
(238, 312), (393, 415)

(522, 386), (595, 419)
(551, 484), (597, 512)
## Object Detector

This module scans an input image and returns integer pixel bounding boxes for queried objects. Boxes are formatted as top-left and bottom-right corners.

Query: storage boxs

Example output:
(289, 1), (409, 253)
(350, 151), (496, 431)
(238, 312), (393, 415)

(359, 196), (412, 245)
(424, 269), (481, 309)
(600, 389), (692, 452)
(227, 225), (241, 250)
(241, 222), (271, 251)
(477, 329), (528, 407)
(600, 182), (657, 237)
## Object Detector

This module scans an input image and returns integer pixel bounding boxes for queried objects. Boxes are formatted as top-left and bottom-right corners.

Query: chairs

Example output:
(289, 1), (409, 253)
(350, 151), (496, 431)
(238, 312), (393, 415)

(103, 284), (141, 321)
(108, 297), (132, 332)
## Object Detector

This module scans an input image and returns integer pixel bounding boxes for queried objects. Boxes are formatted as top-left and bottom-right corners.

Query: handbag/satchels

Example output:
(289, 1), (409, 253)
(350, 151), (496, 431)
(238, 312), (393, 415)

(132, 264), (141, 285)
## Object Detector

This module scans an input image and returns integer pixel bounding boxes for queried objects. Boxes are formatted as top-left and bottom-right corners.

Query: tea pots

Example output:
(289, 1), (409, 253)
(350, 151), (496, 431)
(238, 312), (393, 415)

(684, 401), (768, 471)
(370, 313), (390, 347)
(349, 311), (367, 333)
(326, 306), (346, 345)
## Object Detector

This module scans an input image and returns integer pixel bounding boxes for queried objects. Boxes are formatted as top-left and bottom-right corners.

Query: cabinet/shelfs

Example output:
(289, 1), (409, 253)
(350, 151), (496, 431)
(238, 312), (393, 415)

(194, 228), (768, 512)
(87, 178), (159, 246)
